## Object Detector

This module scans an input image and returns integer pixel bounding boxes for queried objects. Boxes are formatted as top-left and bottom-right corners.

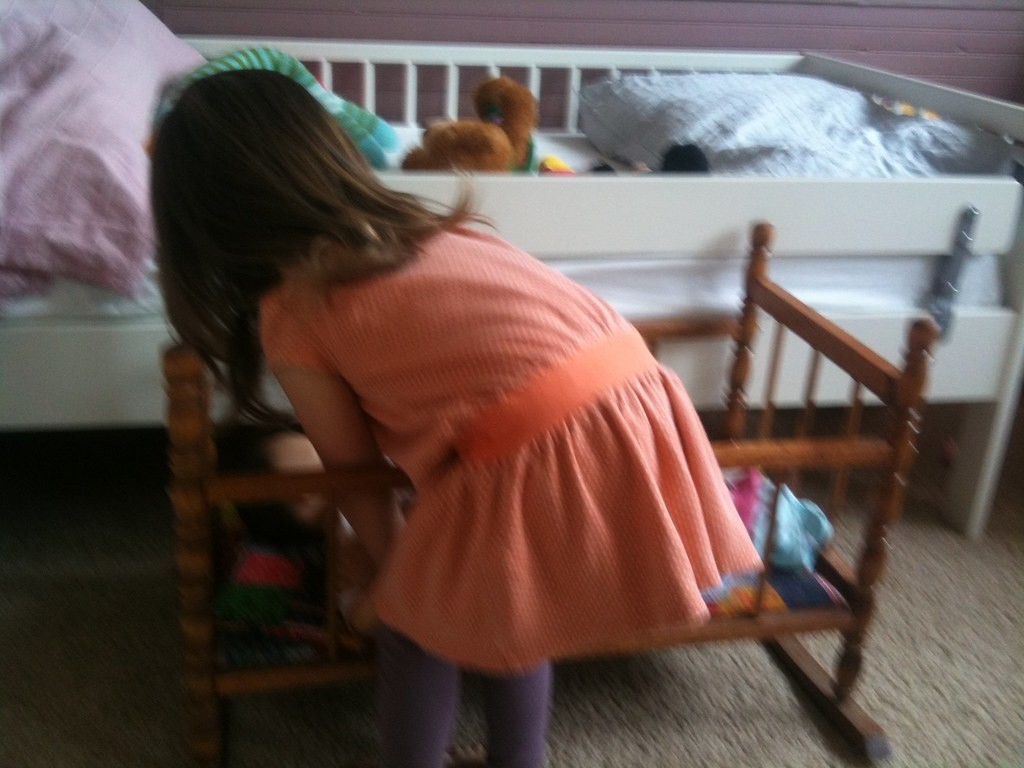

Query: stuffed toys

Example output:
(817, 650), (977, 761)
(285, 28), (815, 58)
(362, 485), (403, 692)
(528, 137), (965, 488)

(400, 77), (538, 176)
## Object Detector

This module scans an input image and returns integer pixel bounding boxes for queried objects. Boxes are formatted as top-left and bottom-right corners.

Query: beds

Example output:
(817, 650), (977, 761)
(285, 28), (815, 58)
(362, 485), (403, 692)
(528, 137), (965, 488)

(162, 219), (942, 768)
(0, 37), (1024, 543)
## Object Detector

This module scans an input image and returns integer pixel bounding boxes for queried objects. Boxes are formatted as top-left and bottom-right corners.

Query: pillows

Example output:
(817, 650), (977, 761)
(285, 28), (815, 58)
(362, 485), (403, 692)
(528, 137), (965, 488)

(581, 73), (1019, 178)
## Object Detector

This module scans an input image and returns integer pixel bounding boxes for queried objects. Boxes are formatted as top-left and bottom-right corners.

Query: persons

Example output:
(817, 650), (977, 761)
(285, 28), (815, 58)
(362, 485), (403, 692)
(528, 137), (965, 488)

(150, 69), (766, 761)
